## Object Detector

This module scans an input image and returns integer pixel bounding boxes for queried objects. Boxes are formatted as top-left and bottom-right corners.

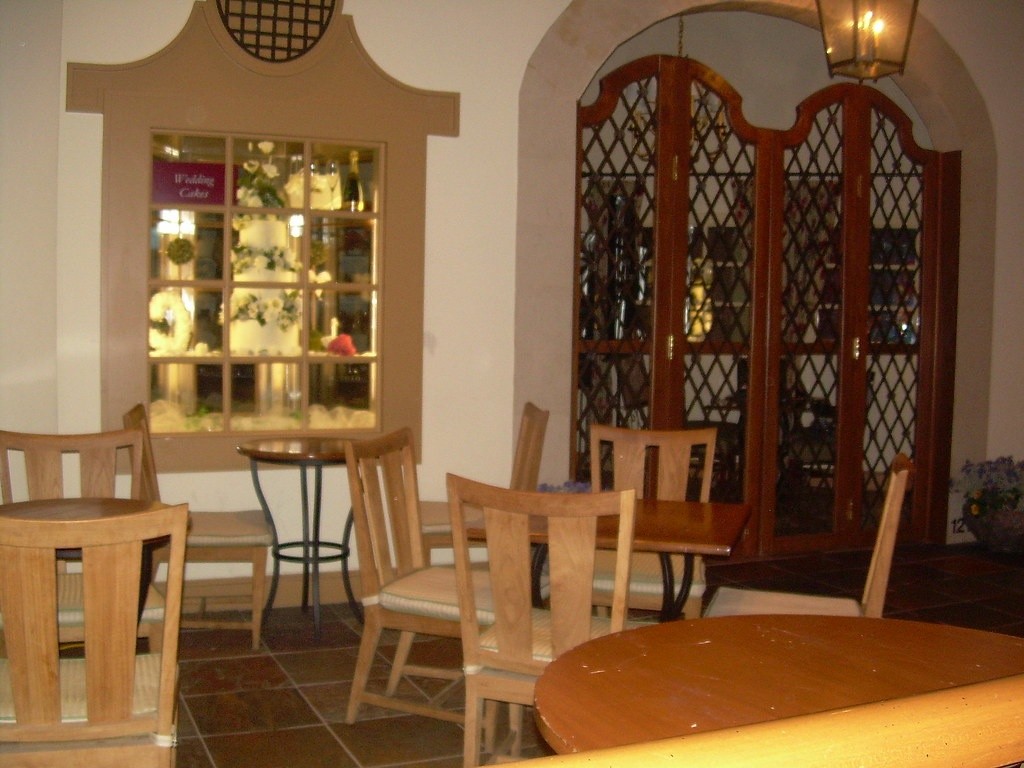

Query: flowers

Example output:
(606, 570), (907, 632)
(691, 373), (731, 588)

(948, 457), (1024, 551)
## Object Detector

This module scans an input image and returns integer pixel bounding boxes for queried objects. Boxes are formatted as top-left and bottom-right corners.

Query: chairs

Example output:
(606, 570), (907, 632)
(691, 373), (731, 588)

(703, 454), (911, 620)
(446, 473), (660, 768)
(590, 424), (718, 620)
(124, 403), (275, 650)
(420, 402), (549, 568)
(0, 503), (189, 768)
(0, 429), (167, 657)
(345, 427), (499, 755)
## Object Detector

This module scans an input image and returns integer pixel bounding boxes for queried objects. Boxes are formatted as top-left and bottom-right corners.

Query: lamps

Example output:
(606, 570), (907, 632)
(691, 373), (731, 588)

(816, 0), (918, 86)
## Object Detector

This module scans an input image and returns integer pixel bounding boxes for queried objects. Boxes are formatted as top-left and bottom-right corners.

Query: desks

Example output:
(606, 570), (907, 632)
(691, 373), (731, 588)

(535, 612), (1024, 754)
(237, 438), (379, 644)
(0, 498), (171, 636)
(465, 499), (752, 622)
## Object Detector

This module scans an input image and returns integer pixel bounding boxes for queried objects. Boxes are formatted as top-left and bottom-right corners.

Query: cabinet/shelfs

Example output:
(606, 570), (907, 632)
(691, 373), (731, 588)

(579, 206), (920, 343)
